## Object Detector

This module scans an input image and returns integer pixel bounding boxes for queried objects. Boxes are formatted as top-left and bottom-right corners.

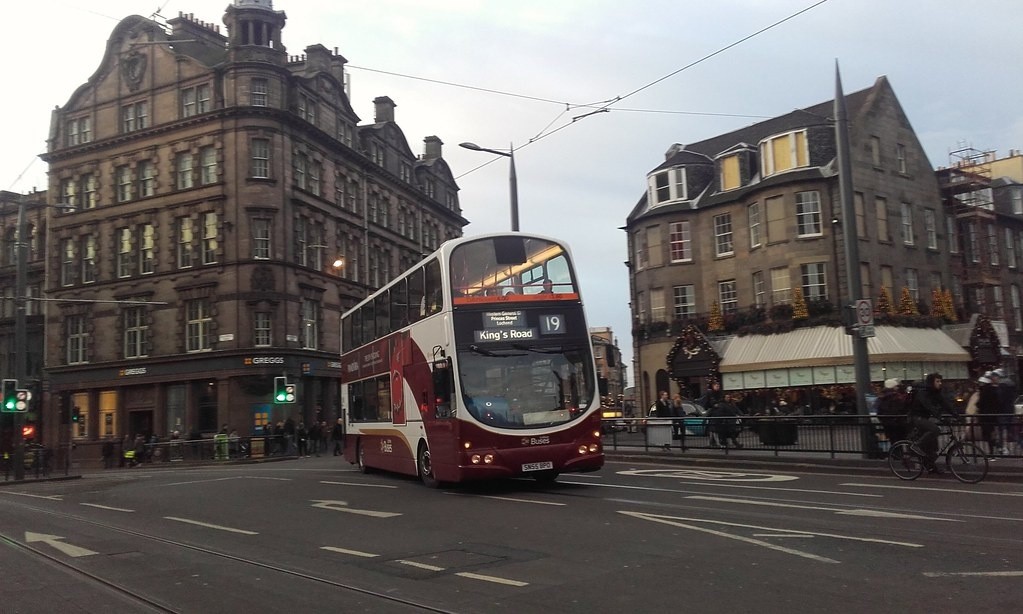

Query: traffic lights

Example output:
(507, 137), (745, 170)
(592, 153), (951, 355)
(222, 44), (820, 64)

(0, 378), (30, 413)
(72, 407), (80, 422)
(23, 425), (34, 436)
(274, 376), (296, 404)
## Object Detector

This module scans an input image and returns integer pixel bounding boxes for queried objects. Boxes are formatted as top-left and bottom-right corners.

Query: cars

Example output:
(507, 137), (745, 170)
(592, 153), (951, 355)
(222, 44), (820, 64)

(639, 400), (743, 438)
(1013, 394), (1023, 416)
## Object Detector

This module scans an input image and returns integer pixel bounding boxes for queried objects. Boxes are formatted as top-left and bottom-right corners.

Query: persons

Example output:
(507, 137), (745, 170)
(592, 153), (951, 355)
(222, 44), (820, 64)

(655, 391), (690, 453)
(170, 430), (180, 441)
(539, 278), (554, 294)
(704, 381), (747, 448)
(874, 373), (967, 477)
(973, 368), (1015, 462)
(218, 424), (239, 459)
(100, 432), (148, 469)
(262, 418), (344, 457)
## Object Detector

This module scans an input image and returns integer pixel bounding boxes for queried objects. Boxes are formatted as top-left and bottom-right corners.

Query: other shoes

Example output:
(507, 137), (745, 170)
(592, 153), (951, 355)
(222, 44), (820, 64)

(910, 443), (927, 456)
(928, 466), (944, 474)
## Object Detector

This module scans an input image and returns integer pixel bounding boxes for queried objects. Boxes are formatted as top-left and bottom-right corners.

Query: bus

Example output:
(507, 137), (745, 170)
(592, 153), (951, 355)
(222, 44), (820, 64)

(339, 232), (617, 490)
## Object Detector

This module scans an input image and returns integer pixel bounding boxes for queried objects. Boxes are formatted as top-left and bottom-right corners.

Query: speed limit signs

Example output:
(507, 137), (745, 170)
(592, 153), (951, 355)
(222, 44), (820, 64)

(858, 301), (872, 324)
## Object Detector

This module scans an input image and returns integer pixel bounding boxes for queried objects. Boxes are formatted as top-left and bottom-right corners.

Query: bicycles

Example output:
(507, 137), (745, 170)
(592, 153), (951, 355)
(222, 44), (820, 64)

(888, 416), (989, 484)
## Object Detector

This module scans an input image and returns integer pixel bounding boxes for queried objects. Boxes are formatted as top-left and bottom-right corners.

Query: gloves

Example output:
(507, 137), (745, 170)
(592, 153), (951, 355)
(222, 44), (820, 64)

(953, 412), (964, 421)
(940, 417), (951, 426)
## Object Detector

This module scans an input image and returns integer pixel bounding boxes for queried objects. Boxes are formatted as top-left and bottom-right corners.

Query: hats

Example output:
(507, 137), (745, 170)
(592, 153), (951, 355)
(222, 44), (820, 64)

(993, 368), (1006, 378)
(979, 371), (993, 383)
(885, 379), (897, 388)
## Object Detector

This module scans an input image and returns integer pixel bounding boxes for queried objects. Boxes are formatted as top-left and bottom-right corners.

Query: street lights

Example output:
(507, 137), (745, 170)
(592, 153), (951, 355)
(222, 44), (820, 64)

(13, 198), (78, 481)
(458, 141), (523, 294)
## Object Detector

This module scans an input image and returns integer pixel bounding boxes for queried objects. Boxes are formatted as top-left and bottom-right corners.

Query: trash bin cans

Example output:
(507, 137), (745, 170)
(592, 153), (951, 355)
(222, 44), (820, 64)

(214, 434), (230, 460)
